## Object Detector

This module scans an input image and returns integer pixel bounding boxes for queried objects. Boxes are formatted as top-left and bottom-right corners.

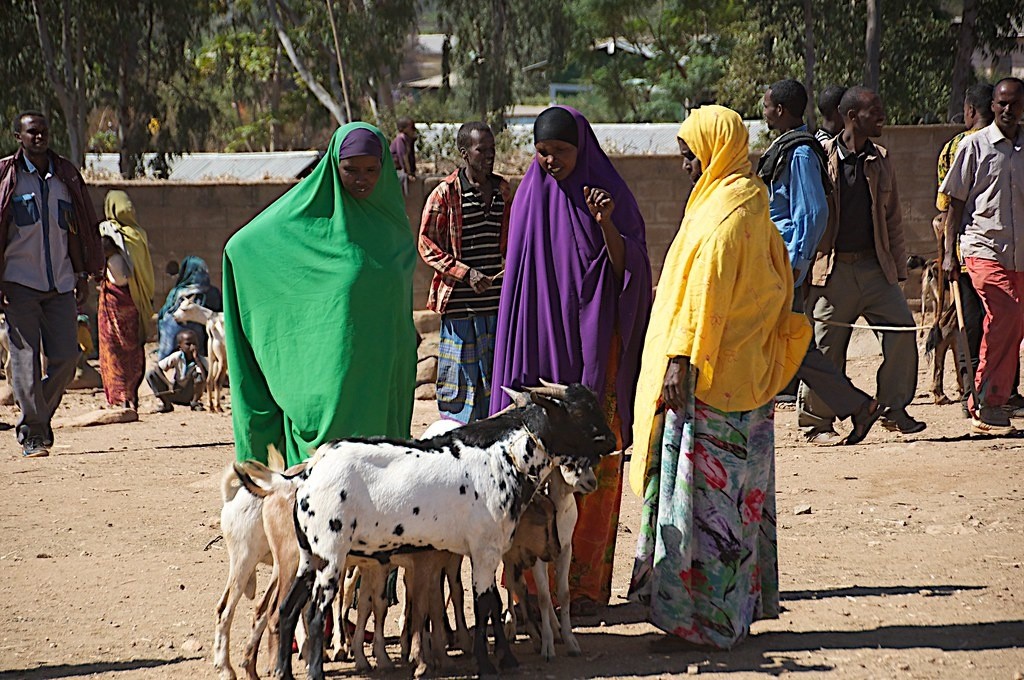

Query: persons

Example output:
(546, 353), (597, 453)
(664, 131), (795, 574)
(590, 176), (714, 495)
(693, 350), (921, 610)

(390, 115), (416, 182)
(755, 78), (1024, 446)
(417, 120), (517, 426)
(488, 106), (655, 622)
(626, 105), (812, 654)
(222, 123), (421, 648)
(0, 112), (223, 458)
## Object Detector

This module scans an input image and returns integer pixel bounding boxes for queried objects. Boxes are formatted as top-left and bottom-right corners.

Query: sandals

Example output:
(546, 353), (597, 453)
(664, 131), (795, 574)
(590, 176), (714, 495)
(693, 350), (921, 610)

(845, 397), (888, 444)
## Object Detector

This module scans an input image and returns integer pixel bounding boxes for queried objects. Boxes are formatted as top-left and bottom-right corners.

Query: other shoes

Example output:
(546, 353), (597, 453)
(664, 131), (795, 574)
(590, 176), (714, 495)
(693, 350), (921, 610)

(886, 408), (926, 432)
(189, 401), (206, 411)
(567, 597), (598, 617)
(804, 426), (844, 445)
(41, 428), (54, 447)
(23, 436), (49, 457)
(151, 402), (175, 414)
(293, 617), (374, 651)
(502, 594), (543, 627)
(1003, 392), (1024, 418)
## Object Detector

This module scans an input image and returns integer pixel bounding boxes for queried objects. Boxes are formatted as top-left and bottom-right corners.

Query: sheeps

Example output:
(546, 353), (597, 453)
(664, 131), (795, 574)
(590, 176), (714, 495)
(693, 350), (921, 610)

(906, 254), (950, 337)
(174, 294), (228, 414)
(214, 376), (617, 679)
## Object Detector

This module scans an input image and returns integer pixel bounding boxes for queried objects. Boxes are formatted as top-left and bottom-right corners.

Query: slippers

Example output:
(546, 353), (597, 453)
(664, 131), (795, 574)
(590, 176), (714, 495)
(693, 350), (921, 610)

(971, 418), (1016, 433)
(775, 401), (797, 412)
